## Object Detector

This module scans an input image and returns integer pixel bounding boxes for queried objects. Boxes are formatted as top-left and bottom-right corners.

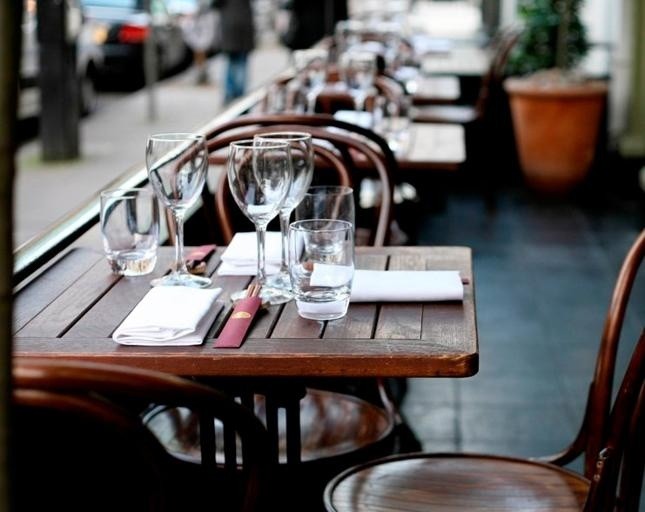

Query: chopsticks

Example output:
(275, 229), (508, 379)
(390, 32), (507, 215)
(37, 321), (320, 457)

(241, 285), (261, 298)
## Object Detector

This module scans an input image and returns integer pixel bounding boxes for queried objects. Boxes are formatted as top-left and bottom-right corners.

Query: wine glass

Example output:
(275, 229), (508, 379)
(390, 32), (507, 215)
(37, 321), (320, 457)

(340, 53), (377, 109)
(292, 49), (328, 117)
(146, 133), (314, 291)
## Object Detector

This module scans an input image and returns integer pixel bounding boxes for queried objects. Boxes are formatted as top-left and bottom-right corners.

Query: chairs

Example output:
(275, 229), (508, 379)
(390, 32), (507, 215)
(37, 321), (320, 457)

(406, 57), (502, 213)
(138, 125), (422, 471)
(11, 357), (275, 511)
(322, 228), (645, 512)
(192, 112), (408, 237)
(264, 25), (417, 120)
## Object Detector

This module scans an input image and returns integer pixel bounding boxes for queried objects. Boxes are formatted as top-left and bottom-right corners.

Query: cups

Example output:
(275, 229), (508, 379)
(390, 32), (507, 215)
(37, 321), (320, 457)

(99, 187), (160, 277)
(295, 184), (356, 266)
(373, 96), (410, 150)
(286, 218), (357, 322)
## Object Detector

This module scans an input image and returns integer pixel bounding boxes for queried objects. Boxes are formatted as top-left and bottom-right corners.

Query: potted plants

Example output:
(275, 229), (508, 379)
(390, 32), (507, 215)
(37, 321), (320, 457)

(503, 1), (618, 196)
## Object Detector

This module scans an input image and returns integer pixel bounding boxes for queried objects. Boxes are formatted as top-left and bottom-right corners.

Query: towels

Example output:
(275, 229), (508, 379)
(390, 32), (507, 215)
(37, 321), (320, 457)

(309, 263), (465, 305)
(217, 232), (290, 276)
(110, 283), (226, 345)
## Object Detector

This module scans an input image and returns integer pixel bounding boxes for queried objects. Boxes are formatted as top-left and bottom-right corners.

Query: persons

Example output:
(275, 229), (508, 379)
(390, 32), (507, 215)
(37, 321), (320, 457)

(210, 0), (254, 107)
